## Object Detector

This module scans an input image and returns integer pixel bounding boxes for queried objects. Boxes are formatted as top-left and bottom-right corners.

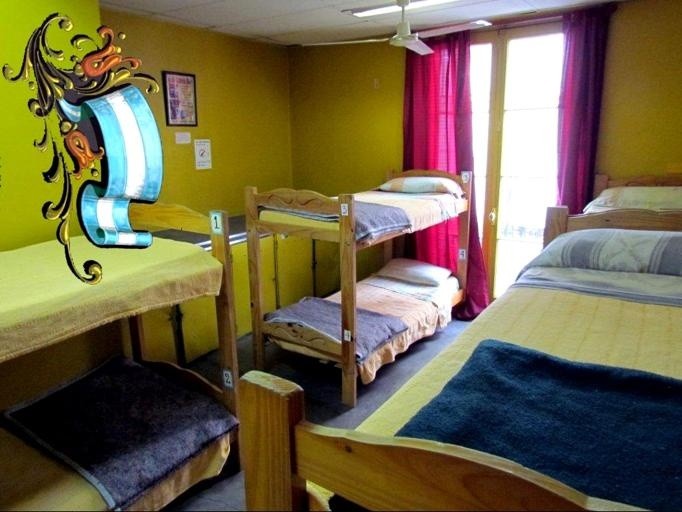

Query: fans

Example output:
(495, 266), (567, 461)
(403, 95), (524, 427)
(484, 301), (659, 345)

(302, 1), (492, 58)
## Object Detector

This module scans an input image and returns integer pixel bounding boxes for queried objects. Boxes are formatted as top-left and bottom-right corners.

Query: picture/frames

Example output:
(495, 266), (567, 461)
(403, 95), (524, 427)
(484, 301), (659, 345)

(161, 70), (197, 127)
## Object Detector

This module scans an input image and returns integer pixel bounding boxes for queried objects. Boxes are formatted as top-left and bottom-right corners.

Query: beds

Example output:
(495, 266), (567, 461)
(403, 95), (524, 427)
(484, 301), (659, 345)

(0, 167), (678, 510)
